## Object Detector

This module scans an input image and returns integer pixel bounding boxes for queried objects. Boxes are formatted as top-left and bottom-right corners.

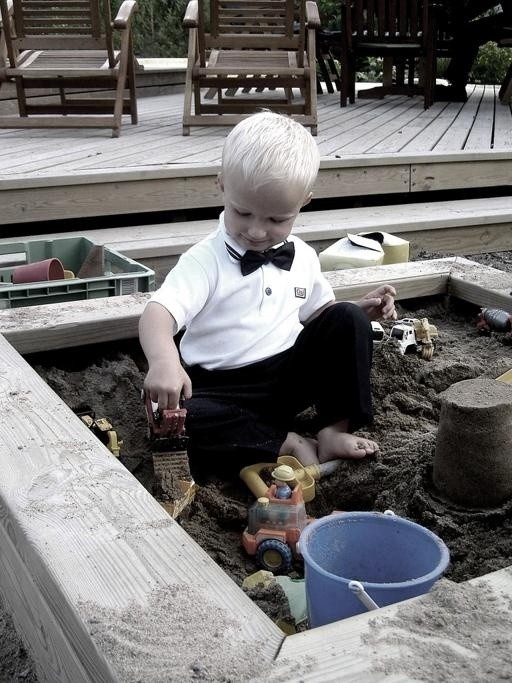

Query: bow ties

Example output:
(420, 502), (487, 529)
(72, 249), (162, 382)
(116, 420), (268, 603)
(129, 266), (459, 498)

(240, 241), (294, 276)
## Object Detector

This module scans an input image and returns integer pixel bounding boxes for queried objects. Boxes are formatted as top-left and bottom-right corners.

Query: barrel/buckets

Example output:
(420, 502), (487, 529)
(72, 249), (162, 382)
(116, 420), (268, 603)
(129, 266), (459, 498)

(295, 510), (451, 630)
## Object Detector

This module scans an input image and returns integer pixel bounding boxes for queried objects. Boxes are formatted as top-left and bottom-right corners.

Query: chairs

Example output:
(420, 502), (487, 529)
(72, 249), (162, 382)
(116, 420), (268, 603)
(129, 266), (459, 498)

(204, 8), (341, 99)
(337, 2), (439, 111)
(0, 2), (145, 139)
(181, 1), (322, 138)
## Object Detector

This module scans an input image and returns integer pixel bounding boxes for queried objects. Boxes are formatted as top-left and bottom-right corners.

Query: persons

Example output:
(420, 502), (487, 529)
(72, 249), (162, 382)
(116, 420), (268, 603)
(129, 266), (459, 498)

(136, 105), (399, 469)
